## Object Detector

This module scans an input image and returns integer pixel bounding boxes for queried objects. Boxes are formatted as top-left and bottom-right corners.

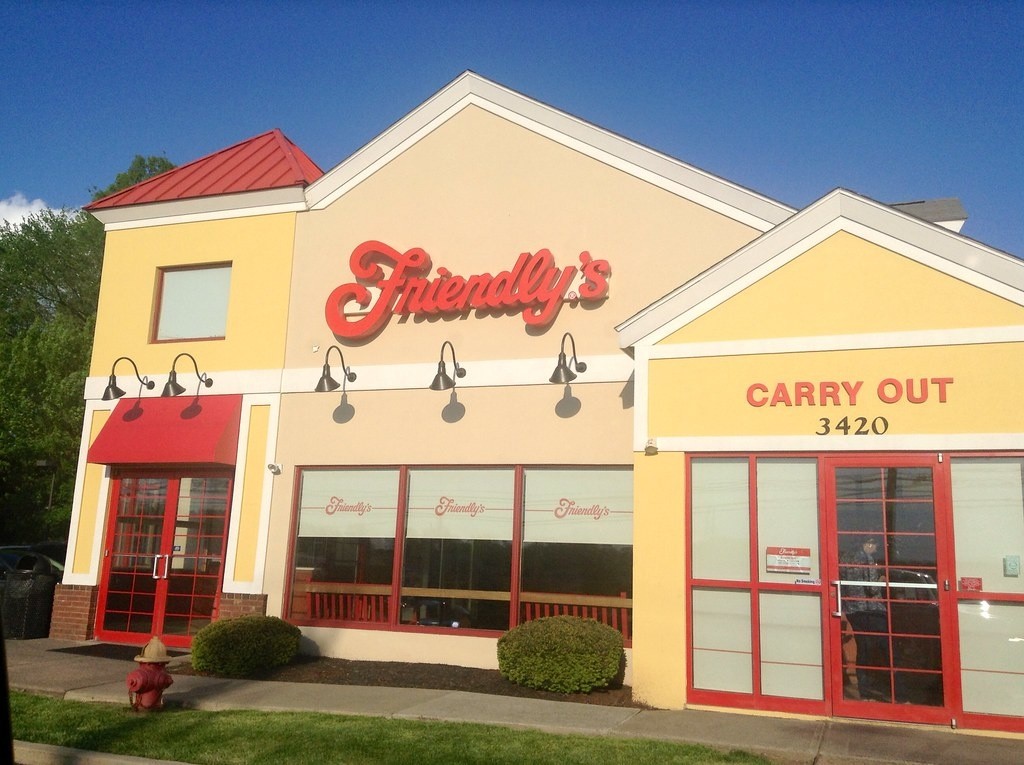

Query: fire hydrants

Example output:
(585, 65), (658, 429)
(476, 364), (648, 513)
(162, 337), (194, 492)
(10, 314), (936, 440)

(126, 636), (173, 709)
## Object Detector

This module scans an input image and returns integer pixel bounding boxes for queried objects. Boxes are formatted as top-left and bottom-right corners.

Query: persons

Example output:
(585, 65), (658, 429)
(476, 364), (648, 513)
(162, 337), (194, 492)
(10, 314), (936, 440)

(839, 534), (888, 700)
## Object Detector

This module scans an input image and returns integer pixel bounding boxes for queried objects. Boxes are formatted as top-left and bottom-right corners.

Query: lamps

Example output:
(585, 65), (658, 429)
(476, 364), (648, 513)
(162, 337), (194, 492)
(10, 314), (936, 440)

(549, 332), (586, 385)
(429, 341), (466, 392)
(160, 353), (213, 398)
(314, 345), (357, 392)
(103, 356), (154, 401)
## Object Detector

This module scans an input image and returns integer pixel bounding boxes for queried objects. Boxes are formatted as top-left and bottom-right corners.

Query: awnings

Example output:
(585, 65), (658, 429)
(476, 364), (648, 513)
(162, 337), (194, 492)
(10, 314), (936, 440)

(87, 394), (243, 466)
